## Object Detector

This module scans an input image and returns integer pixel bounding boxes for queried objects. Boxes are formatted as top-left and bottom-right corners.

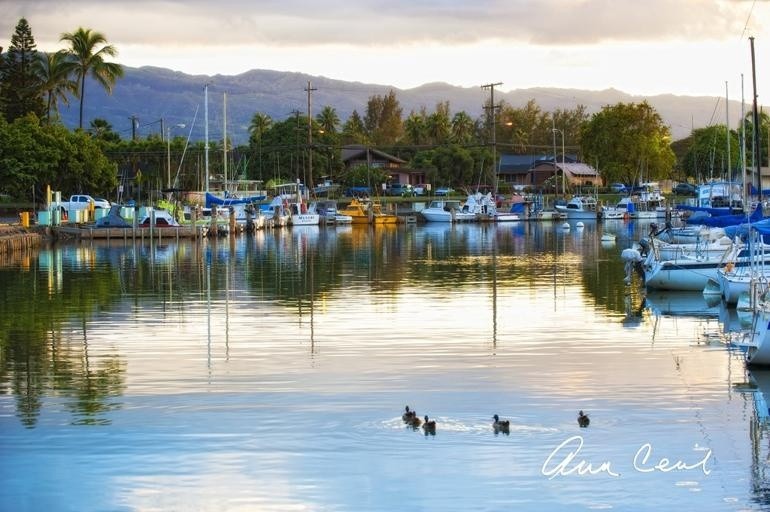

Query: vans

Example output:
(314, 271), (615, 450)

(383, 184), (411, 196)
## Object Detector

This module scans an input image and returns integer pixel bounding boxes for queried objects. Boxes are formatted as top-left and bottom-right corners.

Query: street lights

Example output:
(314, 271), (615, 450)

(552, 128), (566, 192)
(309, 128), (324, 192)
(491, 121), (513, 190)
(166, 121), (185, 189)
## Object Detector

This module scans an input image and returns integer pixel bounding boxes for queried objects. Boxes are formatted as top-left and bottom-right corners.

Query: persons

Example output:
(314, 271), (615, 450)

(119, 196), (136, 206)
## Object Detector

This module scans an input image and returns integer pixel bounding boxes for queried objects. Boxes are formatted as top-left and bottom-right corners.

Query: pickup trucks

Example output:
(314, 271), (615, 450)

(51, 195), (107, 210)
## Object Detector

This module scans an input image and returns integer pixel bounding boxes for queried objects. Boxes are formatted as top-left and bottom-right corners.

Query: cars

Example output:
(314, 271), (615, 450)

(319, 174), (337, 187)
(610, 183), (625, 193)
(434, 186), (455, 196)
(413, 184), (431, 196)
(461, 182), (494, 195)
(95, 198), (117, 208)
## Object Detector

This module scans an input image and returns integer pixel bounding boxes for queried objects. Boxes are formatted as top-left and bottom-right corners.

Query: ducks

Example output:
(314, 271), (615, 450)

(578, 410), (589, 427)
(492, 414), (509, 432)
(403, 406), (412, 424)
(422, 416), (435, 433)
(411, 412), (421, 427)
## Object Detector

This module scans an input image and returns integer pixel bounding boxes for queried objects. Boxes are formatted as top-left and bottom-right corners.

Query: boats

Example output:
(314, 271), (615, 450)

(555, 197), (627, 219)
(461, 192), (520, 221)
(601, 197), (657, 219)
(270, 182), (303, 200)
(421, 200), (476, 223)
(511, 195), (568, 220)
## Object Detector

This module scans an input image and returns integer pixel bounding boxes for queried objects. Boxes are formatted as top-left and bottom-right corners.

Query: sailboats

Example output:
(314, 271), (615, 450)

(157, 83), (267, 205)
(645, 290), (770, 506)
